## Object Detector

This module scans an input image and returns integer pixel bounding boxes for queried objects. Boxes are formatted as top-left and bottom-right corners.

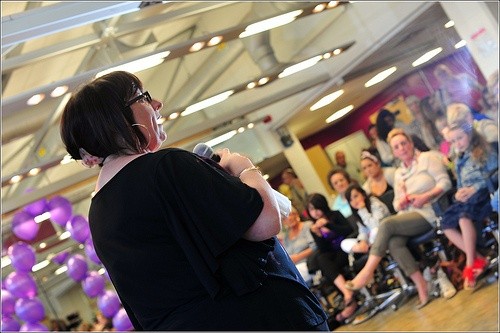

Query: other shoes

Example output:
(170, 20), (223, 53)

(471, 257), (490, 280)
(462, 267), (478, 291)
(335, 304), (361, 322)
(344, 290), (360, 307)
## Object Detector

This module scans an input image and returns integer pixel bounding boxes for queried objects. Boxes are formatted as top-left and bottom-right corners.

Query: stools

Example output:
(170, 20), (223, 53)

(307, 217), (500, 323)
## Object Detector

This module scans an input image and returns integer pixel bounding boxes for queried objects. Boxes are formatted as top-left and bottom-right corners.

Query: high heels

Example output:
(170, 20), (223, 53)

(344, 276), (376, 290)
(416, 281), (434, 310)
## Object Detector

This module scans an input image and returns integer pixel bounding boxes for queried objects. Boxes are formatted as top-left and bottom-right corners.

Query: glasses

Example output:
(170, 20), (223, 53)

(128, 90), (152, 107)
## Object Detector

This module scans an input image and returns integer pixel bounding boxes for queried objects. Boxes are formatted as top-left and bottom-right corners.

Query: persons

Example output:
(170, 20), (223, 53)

(347, 128), (453, 308)
(60, 71), (332, 331)
(277, 63), (500, 328)
(437, 121), (497, 293)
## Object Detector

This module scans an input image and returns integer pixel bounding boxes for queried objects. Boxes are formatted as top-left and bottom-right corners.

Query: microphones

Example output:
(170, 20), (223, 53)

(193, 143), (220, 162)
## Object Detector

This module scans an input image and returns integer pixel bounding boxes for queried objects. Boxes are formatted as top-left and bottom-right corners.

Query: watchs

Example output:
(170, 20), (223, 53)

(403, 193), (412, 208)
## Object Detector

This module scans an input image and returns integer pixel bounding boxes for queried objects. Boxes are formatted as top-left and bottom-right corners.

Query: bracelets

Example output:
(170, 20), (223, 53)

(236, 165), (263, 179)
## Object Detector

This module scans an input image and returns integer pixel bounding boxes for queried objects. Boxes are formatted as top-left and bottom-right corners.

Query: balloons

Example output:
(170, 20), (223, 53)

(1, 195), (135, 332)
